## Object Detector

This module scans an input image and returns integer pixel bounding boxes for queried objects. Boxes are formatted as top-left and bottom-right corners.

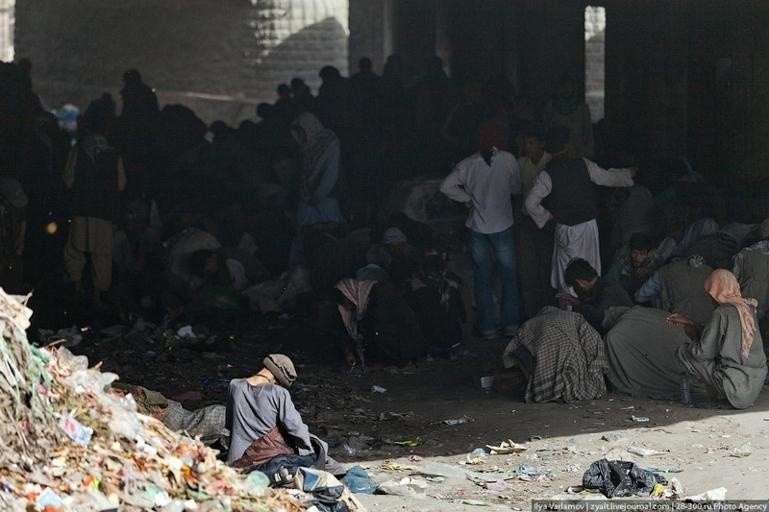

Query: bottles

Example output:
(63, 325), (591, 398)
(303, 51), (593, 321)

(679, 371), (690, 402)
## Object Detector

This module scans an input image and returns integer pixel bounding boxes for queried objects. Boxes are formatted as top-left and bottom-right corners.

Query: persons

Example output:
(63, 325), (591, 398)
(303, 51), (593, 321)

(1, 53), (769, 416)
(225, 353), (343, 478)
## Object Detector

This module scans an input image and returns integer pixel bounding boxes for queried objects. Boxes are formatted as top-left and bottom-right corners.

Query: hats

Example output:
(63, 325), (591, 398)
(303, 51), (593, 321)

(263, 353), (298, 387)
